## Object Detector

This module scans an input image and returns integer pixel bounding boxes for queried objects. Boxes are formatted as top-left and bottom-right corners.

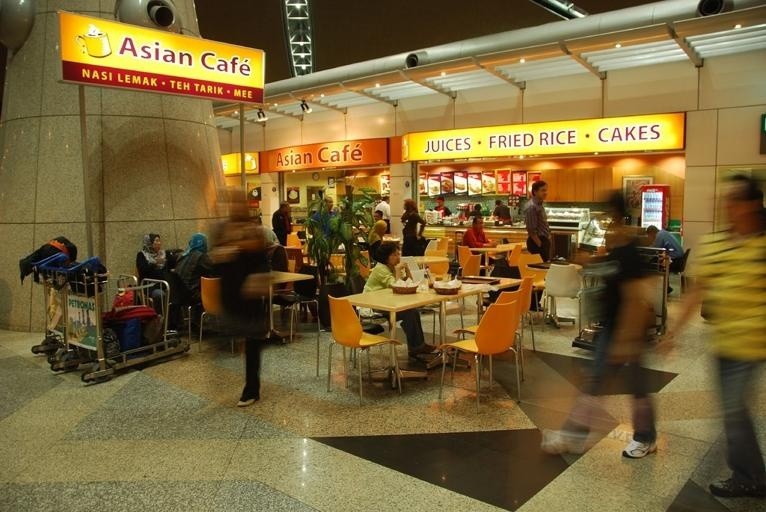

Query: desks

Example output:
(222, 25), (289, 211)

(337, 288), (448, 389)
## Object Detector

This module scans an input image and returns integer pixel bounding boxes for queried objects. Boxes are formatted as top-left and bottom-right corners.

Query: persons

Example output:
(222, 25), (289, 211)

(204, 191), (270, 407)
(655, 173), (766, 499)
(539, 190), (658, 458)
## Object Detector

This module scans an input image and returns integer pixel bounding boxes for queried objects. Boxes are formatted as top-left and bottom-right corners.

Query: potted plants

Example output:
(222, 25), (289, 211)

(298, 186), (345, 330)
(334, 181), (380, 323)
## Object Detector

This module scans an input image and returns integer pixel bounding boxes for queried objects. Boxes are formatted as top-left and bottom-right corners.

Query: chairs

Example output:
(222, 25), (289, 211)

(453, 289), (524, 391)
(440, 300), (520, 415)
(669, 247), (690, 294)
(315, 283), (385, 385)
(544, 264), (579, 328)
(140, 227), (547, 368)
(328, 295), (402, 408)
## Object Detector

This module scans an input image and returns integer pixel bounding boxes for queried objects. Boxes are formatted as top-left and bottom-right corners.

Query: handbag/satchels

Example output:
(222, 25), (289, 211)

(183, 272), (204, 299)
(20, 236), (77, 285)
(100, 315), (164, 357)
(113, 291), (134, 311)
(68, 257), (107, 296)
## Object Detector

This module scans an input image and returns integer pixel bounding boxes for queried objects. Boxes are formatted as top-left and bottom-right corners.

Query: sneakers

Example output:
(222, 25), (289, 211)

(422, 344), (436, 353)
(541, 438), (584, 455)
(710, 478), (766, 498)
(622, 440), (657, 458)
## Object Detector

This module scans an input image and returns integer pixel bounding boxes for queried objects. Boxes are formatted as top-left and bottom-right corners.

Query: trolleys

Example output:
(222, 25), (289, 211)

(571, 245), (674, 358)
(25, 252), (138, 365)
(39, 253), (190, 382)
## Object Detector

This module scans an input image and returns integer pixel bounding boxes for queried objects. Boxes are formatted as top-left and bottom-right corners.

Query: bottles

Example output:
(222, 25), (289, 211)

(644, 192), (662, 229)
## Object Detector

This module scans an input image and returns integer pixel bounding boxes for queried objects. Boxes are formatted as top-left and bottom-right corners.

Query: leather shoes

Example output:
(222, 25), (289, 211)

(237, 399), (255, 407)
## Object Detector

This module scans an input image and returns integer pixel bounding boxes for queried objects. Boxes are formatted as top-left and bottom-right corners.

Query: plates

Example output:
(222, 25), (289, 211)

(419, 174), (496, 194)
(385, 182), (390, 191)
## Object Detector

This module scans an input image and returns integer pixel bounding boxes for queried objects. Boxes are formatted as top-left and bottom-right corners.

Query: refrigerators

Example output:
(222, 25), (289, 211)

(641, 184), (671, 230)
